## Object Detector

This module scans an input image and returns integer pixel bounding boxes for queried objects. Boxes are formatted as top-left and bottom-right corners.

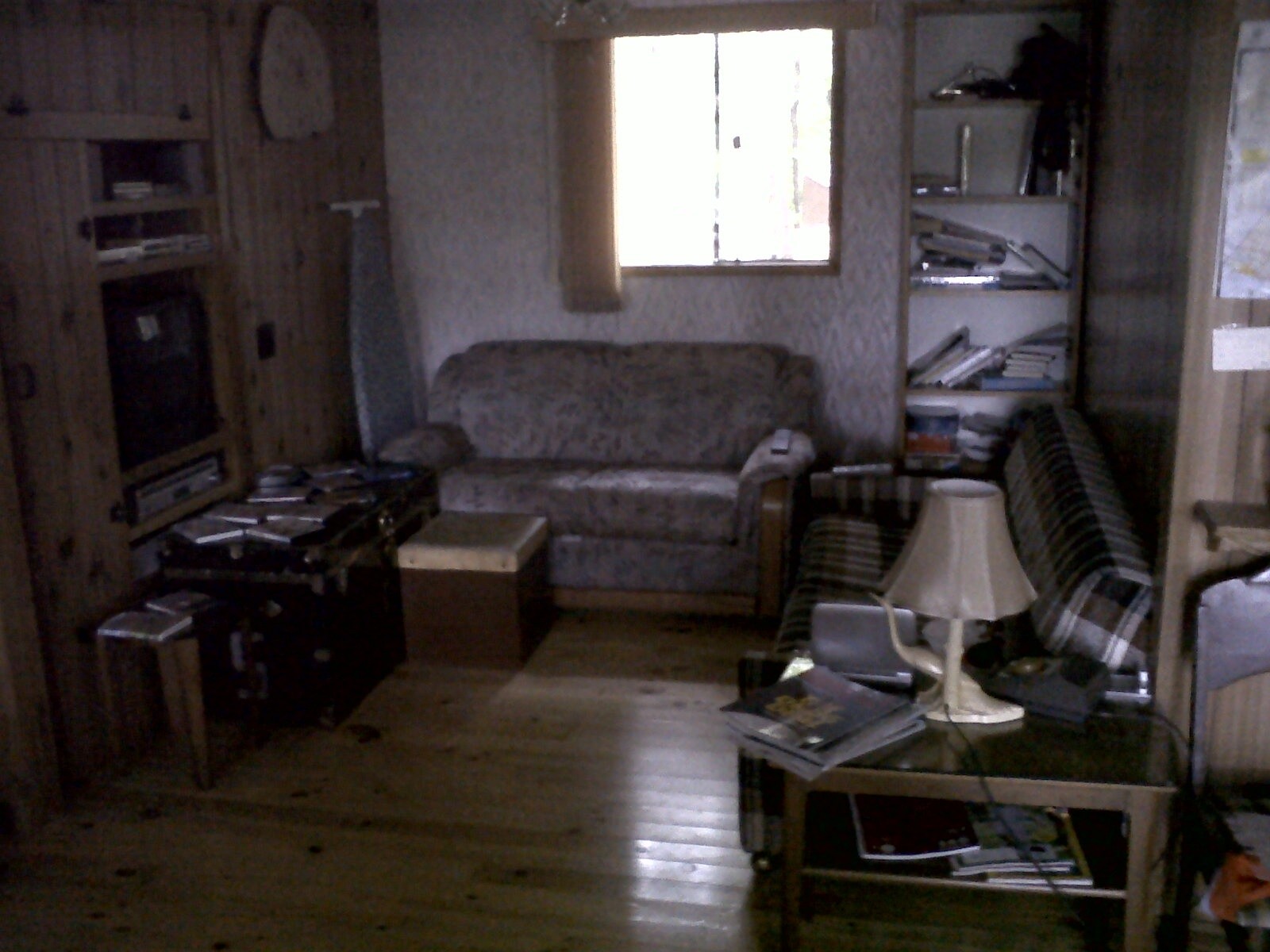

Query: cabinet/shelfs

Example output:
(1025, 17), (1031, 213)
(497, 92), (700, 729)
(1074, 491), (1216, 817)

(895, 5), (1098, 466)
(4, 3), (389, 856)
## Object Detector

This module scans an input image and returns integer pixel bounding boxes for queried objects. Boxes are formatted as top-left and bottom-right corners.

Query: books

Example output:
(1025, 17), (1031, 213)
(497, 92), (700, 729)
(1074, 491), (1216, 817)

(314, 484), (377, 505)
(305, 473), (357, 493)
(908, 105), (1082, 466)
(98, 608), (193, 644)
(359, 461), (413, 483)
(147, 590), (219, 619)
(846, 797), (984, 859)
(247, 484), (312, 504)
(303, 458), (358, 479)
(944, 804), (1096, 890)
(246, 515), (326, 547)
(264, 503), (346, 524)
(711, 661), (942, 787)
(172, 513), (244, 543)
(204, 502), (266, 524)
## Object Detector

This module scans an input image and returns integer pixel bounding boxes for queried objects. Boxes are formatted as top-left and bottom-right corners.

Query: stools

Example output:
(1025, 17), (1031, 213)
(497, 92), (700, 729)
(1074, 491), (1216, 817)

(394, 506), (550, 670)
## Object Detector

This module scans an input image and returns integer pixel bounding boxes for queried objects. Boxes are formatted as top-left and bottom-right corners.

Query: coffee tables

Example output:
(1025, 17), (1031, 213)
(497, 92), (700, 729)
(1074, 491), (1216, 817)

(162, 464), (439, 724)
(755, 671), (1190, 947)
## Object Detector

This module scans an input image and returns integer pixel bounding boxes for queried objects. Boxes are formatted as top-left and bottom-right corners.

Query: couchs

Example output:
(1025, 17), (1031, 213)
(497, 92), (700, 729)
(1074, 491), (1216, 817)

(377, 336), (823, 621)
(730, 400), (1156, 876)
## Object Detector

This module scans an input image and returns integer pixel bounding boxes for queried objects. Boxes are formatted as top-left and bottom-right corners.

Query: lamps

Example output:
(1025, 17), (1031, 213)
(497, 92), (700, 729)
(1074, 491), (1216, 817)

(871, 475), (1040, 728)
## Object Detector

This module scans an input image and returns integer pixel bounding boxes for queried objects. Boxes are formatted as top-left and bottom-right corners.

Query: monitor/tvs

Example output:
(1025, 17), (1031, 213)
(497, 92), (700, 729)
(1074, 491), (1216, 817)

(98, 280), (217, 459)
